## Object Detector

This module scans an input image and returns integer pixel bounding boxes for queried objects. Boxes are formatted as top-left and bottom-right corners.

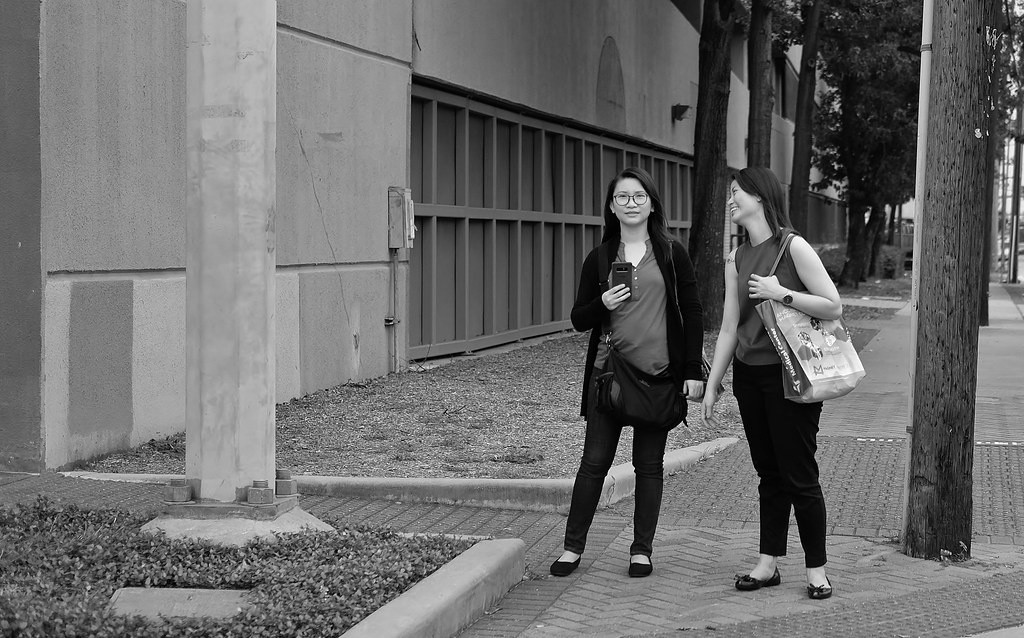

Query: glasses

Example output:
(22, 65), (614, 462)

(613, 192), (648, 206)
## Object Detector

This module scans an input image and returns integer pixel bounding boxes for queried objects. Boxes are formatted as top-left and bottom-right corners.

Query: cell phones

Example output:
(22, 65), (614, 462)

(612, 262), (633, 302)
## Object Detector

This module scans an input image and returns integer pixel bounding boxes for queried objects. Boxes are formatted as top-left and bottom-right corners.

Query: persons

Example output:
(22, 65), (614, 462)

(550, 166), (703, 578)
(701, 166), (843, 600)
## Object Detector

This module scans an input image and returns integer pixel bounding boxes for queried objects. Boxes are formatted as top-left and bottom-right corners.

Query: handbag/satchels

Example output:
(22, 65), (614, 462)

(755, 235), (866, 403)
(595, 346), (689, 431)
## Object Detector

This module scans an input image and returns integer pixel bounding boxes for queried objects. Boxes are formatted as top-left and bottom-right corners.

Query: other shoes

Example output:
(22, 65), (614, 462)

(734, 565), (781, 591)
(628, 554), (653, 577)
(807, 575), (832, 599)
(550, 549), (581, 576)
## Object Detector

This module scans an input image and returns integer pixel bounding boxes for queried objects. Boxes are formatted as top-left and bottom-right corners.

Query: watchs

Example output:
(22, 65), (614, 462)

(783, 289), (793, 307)
